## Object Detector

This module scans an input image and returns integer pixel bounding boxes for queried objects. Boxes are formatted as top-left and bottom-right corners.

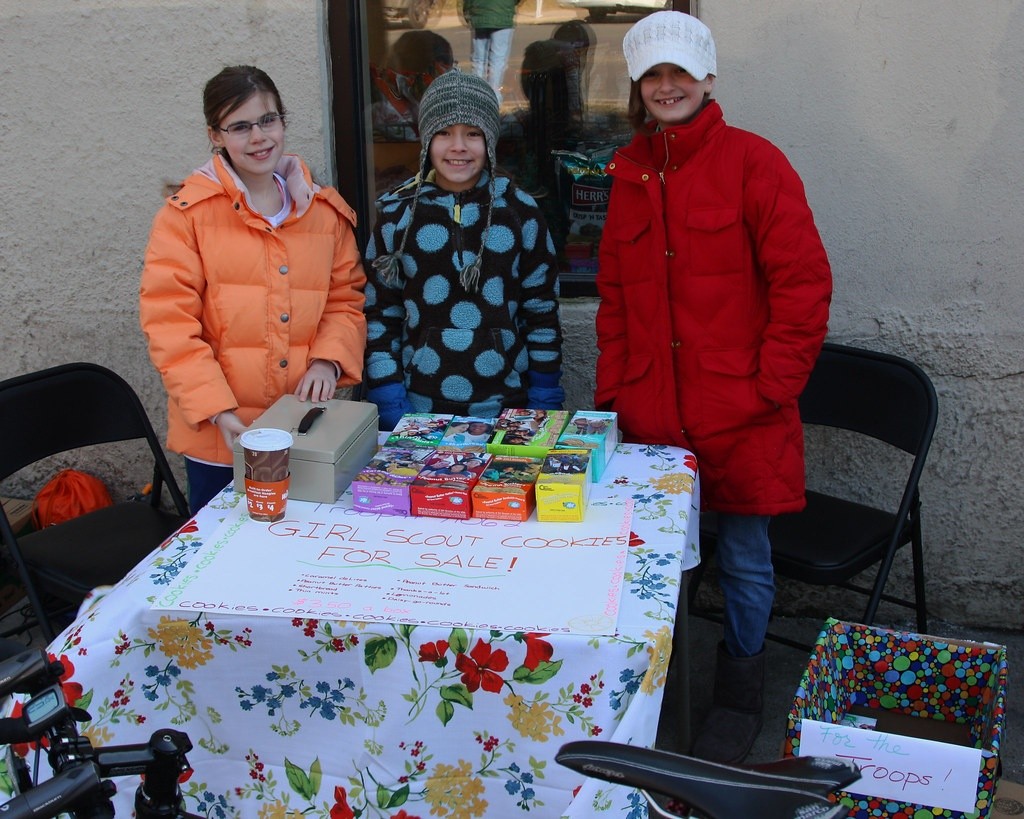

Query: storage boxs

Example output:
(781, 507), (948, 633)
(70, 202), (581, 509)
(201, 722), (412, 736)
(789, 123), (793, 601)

(231, 393), (382, 504)
(779, 617), (1006, 819)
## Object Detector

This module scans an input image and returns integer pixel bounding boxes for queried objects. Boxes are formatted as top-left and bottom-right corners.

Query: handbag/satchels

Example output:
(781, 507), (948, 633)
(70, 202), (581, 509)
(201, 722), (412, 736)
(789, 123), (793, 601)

(38, 469), (116, 532)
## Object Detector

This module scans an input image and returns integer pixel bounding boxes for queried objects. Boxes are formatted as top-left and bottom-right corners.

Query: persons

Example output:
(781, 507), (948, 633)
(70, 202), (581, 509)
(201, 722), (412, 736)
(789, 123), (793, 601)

(361, 68), (567, 433)
(462, 0), (521, 109)
(595, 9), (836, 768)
(138, 64), (369, 518)
(496, 19), (591, 189)
(372, 30), (454, 142)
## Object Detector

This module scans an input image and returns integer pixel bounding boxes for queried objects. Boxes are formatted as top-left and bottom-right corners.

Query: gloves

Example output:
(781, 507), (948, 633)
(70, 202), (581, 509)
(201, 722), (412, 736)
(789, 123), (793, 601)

(365, 385), (415, 430)
(527, 371), (566, 411)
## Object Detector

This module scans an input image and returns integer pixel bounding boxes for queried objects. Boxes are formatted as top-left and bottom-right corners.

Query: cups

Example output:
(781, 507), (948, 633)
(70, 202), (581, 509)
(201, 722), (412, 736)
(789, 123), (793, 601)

(238, 427), (296, 523)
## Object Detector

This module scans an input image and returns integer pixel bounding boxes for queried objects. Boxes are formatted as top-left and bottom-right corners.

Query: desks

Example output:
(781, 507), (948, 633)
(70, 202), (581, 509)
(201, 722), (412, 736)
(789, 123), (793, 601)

(51, 432), (703, 819)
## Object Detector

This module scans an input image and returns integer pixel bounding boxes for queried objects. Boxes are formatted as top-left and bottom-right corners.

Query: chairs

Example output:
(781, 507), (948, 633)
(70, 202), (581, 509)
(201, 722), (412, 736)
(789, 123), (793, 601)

(689, 340), (940, 657)
(1, 361), (190, 645)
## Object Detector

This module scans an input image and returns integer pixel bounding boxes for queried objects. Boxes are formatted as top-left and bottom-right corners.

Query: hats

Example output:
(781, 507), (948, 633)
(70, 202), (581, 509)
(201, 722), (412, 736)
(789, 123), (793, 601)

(623, 11), (716, 81)
(371, 69), (500, 292)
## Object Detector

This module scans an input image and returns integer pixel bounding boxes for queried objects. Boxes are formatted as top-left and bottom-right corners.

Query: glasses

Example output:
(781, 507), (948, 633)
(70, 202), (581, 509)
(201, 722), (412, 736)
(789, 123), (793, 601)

(218, 114), (285, 139)
